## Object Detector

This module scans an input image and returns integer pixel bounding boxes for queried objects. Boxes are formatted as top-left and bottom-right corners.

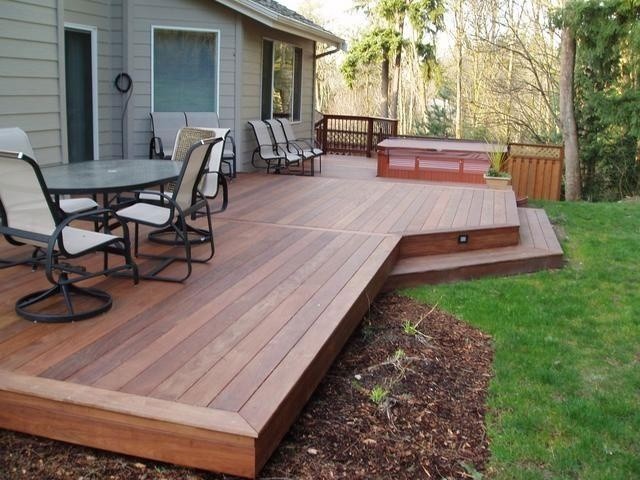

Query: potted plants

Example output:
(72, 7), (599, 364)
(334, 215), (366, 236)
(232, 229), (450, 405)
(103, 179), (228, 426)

(482, 135), (518, 186)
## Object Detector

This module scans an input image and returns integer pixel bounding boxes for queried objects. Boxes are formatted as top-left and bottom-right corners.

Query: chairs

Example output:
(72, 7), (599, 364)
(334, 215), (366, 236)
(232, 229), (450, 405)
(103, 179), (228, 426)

(185, 112), (236, 178)
(111, 137), (223, 283)
(264, 119), (316, 176)
(247, 120), (301, 174)
(149, 111), (187, 160)
(0, 127), (99, 273)
(0, 150), (133, 323)
(135, 128), (230, 221)
(276, 118), (325, 173)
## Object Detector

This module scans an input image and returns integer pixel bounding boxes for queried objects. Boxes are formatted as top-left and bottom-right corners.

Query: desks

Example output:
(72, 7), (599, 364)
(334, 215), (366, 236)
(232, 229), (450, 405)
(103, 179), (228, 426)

(37, 158), (185, 277)
